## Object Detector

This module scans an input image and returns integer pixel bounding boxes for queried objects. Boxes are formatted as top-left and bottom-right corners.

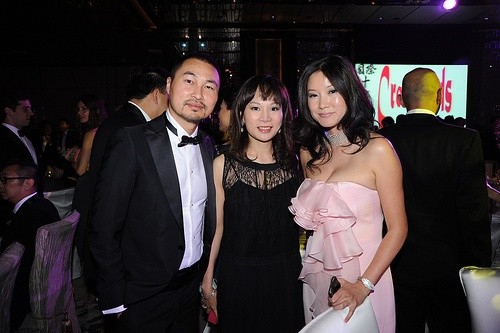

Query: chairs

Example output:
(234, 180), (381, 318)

(21, 210), (81, 333)
(0, 241), (25, 333)
(459, 265), (500, 333)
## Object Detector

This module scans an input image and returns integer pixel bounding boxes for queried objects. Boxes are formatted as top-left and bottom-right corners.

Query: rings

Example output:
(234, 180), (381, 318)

(340, 304), (344, 309)
(202, 304), (207, 309)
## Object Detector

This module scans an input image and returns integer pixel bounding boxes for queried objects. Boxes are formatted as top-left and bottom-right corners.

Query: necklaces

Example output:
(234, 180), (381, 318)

(326, 131), (350, 147)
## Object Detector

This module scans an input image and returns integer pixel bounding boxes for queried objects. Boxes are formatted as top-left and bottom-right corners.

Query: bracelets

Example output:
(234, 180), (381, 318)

(199, 278), (217, 300)
(358, 277), (375, 291)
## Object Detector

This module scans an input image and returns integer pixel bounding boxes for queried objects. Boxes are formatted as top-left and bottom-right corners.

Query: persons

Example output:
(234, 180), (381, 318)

(219, 94), (239, 139)
(82, 52), (223, 333)
(197, 73), (305, 333)
(0, 70), (100, 190)
(0, 162), (61, 333)
(377, 68), (491, 332)
(288, 56), (409, 333)
(86, 67), (169, 218)
(379, 114), (500, 203)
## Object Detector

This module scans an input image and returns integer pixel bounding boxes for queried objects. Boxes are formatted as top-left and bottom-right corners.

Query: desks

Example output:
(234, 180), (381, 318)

(43, 188), (83, 280)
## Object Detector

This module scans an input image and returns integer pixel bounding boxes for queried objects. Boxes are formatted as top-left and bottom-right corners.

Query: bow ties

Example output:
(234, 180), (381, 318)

(166, 117), (203, 147)
(17, 129), (25, 137)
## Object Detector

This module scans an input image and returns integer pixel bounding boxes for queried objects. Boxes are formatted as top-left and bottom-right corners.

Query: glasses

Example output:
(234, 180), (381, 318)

(0, 176), (29, 184)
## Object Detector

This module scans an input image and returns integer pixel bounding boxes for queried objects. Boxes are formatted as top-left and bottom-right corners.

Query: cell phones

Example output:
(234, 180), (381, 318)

(329, 276), (340, 298)
